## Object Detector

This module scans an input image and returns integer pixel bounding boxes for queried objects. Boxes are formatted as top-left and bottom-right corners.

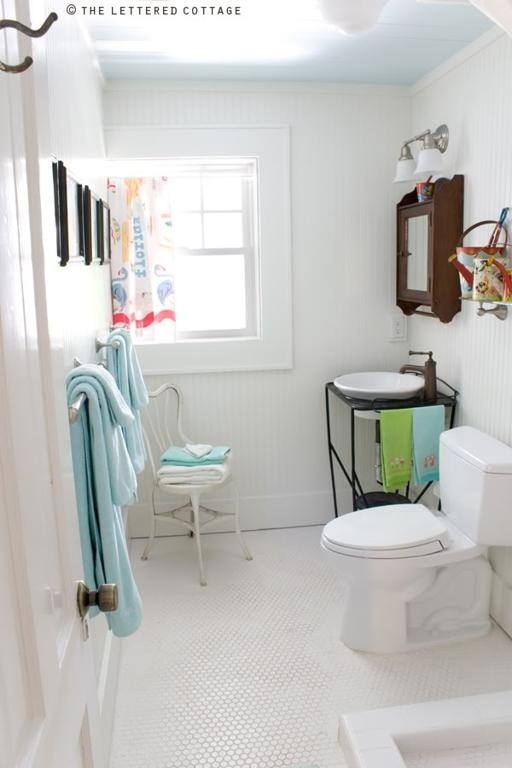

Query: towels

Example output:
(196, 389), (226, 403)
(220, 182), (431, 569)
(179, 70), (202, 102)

(104, 328), (149, 474)
(157, 466), (232, 485)
(378, 402), (446, 492)
(65, 362), (143, 640)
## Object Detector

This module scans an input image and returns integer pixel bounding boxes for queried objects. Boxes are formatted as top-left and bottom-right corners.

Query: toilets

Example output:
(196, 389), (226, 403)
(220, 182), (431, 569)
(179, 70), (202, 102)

(321, 424), (512, 651)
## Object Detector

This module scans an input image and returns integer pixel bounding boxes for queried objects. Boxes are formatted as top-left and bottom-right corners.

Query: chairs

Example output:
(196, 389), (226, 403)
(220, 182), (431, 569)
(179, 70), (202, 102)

(131, 383), (253, 587)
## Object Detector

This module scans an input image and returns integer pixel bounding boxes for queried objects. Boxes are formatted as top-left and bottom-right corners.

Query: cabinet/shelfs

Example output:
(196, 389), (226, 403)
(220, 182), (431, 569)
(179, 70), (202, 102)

(394, 174), (464, 324)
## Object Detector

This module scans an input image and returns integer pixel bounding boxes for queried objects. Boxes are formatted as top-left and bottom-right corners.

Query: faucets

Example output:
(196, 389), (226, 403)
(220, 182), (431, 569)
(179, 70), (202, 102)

(399, 350), (436, 400)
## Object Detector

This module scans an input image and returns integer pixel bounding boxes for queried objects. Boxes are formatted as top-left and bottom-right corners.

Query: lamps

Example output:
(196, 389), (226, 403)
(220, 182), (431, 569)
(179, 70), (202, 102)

(394, 121), (450, 177)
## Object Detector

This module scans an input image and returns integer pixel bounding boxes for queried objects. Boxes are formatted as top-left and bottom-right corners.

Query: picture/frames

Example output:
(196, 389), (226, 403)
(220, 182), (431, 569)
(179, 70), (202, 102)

(49, 157), (112, 266)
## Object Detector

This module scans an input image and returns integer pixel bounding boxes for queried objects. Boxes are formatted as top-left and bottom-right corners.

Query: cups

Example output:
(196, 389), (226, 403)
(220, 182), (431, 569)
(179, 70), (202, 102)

(416, 182), (434, 202)
(472, 259), (508, 300)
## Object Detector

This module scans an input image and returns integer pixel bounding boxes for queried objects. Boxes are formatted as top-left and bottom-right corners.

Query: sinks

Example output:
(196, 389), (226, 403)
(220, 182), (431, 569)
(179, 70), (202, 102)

(334, 372), (424, 403)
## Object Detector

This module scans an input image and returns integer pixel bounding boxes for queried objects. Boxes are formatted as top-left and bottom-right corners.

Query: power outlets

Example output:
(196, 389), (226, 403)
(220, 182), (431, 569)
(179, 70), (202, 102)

(387, 315), (408, 341)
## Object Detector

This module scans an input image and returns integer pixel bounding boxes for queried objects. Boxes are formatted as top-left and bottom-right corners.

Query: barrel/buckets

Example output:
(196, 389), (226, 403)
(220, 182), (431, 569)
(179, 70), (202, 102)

(453, 220), (507, 299)
